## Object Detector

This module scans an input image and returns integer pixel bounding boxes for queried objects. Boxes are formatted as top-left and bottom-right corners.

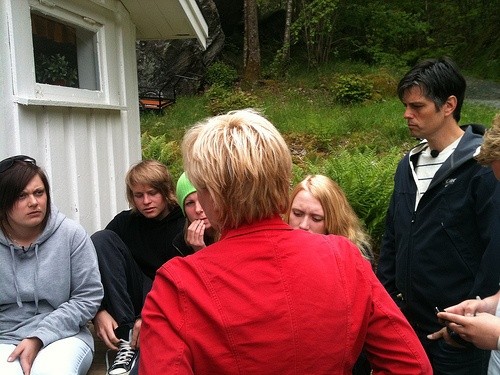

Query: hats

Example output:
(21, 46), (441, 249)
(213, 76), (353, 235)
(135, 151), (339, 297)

(176, 169), (198, 218)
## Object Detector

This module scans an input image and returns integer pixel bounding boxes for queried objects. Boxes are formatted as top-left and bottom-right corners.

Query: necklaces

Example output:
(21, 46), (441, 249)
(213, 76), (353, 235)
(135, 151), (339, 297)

(7, 232), (20, 247)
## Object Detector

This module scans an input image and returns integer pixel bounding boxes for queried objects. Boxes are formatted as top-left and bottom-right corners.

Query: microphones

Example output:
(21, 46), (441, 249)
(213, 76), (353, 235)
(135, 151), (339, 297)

(431, 149), (439, 157)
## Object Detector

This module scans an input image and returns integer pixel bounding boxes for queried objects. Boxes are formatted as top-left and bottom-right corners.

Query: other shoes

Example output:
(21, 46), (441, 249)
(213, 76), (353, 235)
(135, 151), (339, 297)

(107, 329), (138, 375)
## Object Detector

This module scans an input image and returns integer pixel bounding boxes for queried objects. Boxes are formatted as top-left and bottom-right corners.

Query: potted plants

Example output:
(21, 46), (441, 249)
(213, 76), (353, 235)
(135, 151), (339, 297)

(36, 46), (78, 87)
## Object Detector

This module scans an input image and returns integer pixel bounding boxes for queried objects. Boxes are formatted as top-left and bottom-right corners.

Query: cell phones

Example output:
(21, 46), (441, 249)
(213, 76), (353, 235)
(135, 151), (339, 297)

(434, 306), (445, 315)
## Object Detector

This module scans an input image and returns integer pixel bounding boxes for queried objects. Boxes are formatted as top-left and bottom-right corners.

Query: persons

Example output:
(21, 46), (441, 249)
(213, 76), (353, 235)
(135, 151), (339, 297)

(437, 111), (500, 375)
(173, 171), (217, 258)
(352, 55), (500, 375)
(138, 107), (433, 375)
(90, 160), (185, 375)
(0, 155), (104, 375)
(283, 175), (374, 263)
